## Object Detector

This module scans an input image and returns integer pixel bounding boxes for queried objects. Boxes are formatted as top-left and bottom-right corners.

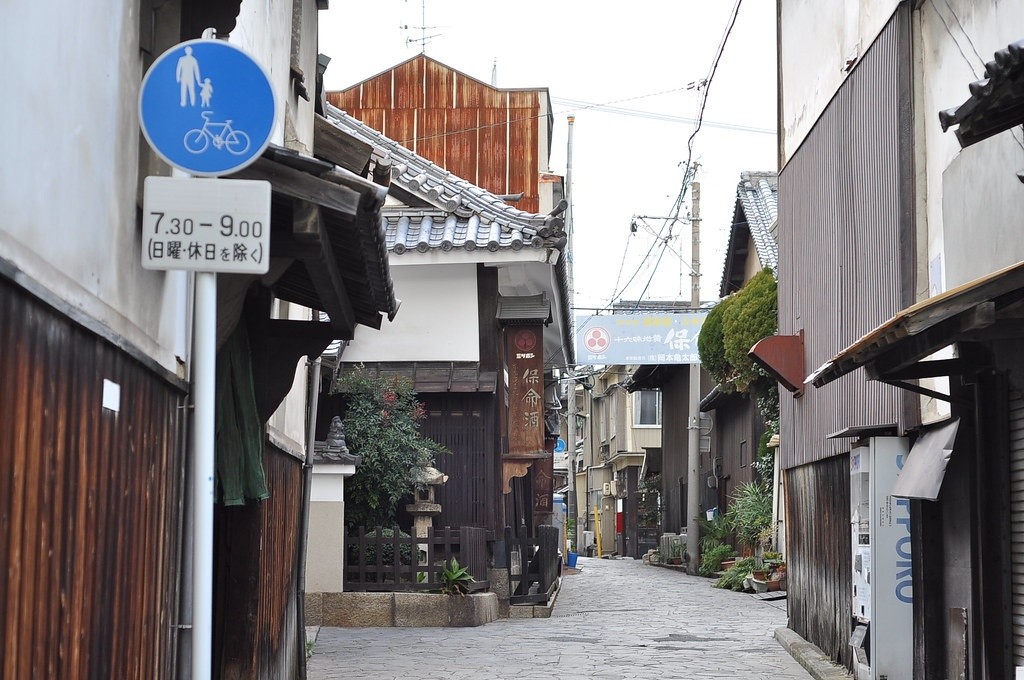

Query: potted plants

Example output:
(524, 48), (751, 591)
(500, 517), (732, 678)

(779, 572), (787, 591)
(670, 544), (684, 565)
(765, 552), (783, 573)
(752, 565), (766, 581)
(765, 572), (779, 591)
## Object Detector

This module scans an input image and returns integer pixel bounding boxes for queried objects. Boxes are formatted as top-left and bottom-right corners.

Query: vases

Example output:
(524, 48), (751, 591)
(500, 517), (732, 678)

(720, 561), (735, 571)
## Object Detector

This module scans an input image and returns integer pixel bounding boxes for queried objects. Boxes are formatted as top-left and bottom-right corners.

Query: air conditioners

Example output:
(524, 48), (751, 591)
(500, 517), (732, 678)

(583, 531), (594, 549)
(603, 482), (611, 496)
